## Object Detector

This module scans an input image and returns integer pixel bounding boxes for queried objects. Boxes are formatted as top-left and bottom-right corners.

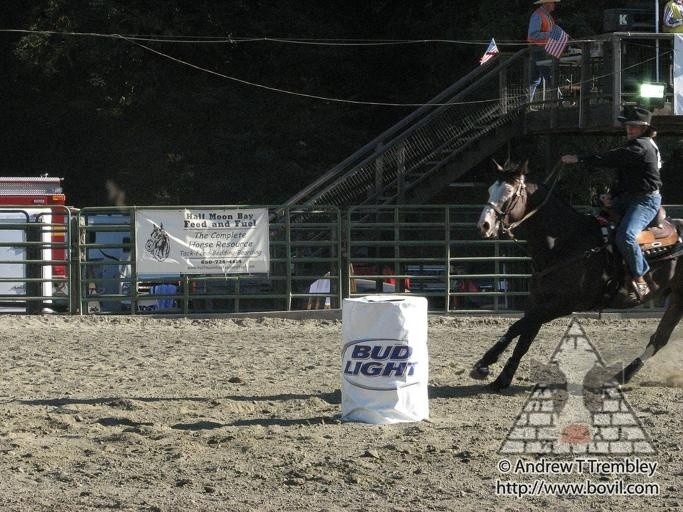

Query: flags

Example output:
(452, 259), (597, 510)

(543, 23), (570, 60)
(478, 37), (498, 67)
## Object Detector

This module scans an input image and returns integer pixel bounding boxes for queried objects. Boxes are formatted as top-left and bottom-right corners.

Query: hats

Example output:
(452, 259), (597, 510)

(618, 108), (652, 127)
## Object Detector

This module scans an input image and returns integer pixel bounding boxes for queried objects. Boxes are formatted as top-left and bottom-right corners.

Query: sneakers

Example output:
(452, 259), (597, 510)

(625, 283), (650, 303)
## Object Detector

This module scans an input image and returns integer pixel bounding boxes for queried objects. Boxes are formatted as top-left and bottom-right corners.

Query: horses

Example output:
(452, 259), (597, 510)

(469, 157), (683, 393)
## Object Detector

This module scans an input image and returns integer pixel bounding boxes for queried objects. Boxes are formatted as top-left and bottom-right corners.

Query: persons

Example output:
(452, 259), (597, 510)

(662, 0), (683, 102)
(561, 106), (662, 306)
(520, 1), (577, 112)
